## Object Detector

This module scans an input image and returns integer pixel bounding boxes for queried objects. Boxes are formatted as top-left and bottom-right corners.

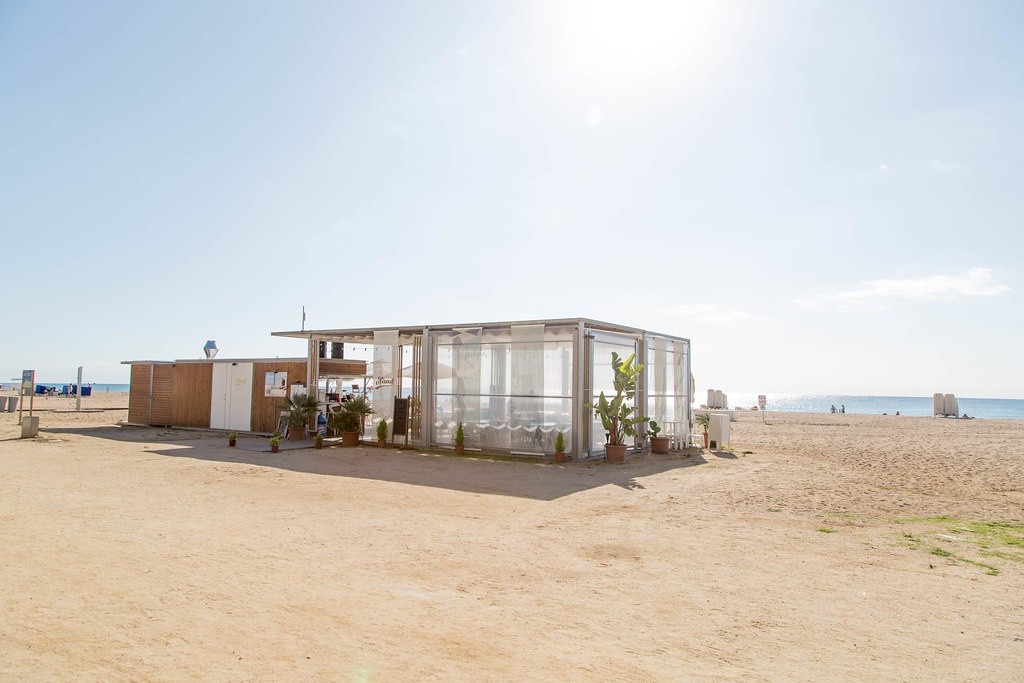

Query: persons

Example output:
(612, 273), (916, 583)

(841, 405), (845, 414)
(830, 405), (838, 414)
(66, 384), (75, 399)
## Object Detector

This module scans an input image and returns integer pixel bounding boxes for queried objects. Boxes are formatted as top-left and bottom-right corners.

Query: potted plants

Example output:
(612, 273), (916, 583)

(280, 392), (323, 441)
(695, 413), (710, 448)
(228, 430), (237, 446)
(314, 434), (324, 449)
(642, 420), (670, 454)
(454, 421), (465, 454)
(376, 419), (388, 447)
(329, 397), (379, 446)
(581, 351), (652, 464)
(554, 431), (565, 463)
(269, 438), (281, 452)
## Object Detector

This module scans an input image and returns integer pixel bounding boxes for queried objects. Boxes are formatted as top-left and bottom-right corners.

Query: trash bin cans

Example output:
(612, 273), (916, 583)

(0, 396), (7, 412)
(8, 396), (19, 412)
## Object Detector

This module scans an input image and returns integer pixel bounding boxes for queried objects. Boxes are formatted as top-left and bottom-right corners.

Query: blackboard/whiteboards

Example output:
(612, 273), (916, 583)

(391, 395), (410, 449)
(276, 410), (291, 439)
